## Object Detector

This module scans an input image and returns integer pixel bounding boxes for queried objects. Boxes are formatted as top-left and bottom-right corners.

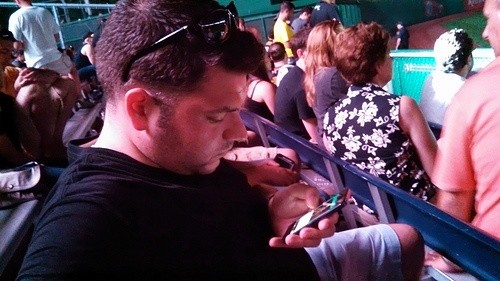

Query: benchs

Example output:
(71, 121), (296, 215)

(242, 106), (500, 281)
(0, 101), (103, 281)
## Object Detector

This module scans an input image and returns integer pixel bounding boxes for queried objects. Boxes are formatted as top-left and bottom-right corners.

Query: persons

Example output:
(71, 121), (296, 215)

(323, 22), (437, 215)
(245, 44), (277, 122)
(0, 0), (301, 281)
(274, 1), (297, 65)
(268, 42), (295, 87)
(305, 20), (347, 149)
(20, 0), (425, 281)
(310, 0), (343, 28)
(396, 22), (410, 50)
(421, 28), (473, 125)
(292, 7), (312, 32)
(8, 0), (95, 109)
(425, 0), (500, 281)
(274, 27), (318, 145)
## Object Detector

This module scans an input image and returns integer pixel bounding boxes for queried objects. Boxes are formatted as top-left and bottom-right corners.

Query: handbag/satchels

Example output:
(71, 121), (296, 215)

(1, 162), (47, 211)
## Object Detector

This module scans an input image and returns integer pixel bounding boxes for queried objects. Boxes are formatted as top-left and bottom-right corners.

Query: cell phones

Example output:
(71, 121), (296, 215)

(282, 188), (352, 243)
(273, 153), (296, 169)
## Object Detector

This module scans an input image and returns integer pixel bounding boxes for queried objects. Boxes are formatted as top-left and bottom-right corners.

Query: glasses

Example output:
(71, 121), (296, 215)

(121, 0), (241, 83)
